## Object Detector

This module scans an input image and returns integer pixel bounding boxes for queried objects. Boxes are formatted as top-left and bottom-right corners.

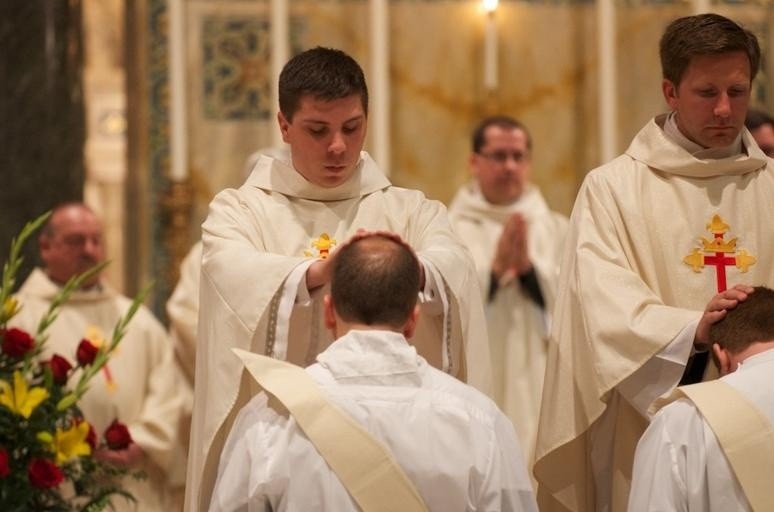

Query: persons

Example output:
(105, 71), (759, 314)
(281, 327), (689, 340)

(742, 108), (774, 158)
(626, 285), (774, 510)
(532, 14), (774, 512)
(207, 232), (540, 512)
(6, 203), (194, 512)
(447, 115), (569, 464)
(184, 54), (491, 512)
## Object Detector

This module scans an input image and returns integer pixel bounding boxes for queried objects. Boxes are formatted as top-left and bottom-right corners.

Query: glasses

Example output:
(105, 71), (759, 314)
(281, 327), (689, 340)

(479, 148), (526, 162)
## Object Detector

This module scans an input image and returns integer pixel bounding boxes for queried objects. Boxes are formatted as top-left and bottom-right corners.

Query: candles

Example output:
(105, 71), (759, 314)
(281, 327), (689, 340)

(483, 6), (499, 95)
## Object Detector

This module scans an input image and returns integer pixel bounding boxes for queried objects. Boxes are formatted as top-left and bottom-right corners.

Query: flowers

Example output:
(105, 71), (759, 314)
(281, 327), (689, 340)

(0, 211), (154, 512)
